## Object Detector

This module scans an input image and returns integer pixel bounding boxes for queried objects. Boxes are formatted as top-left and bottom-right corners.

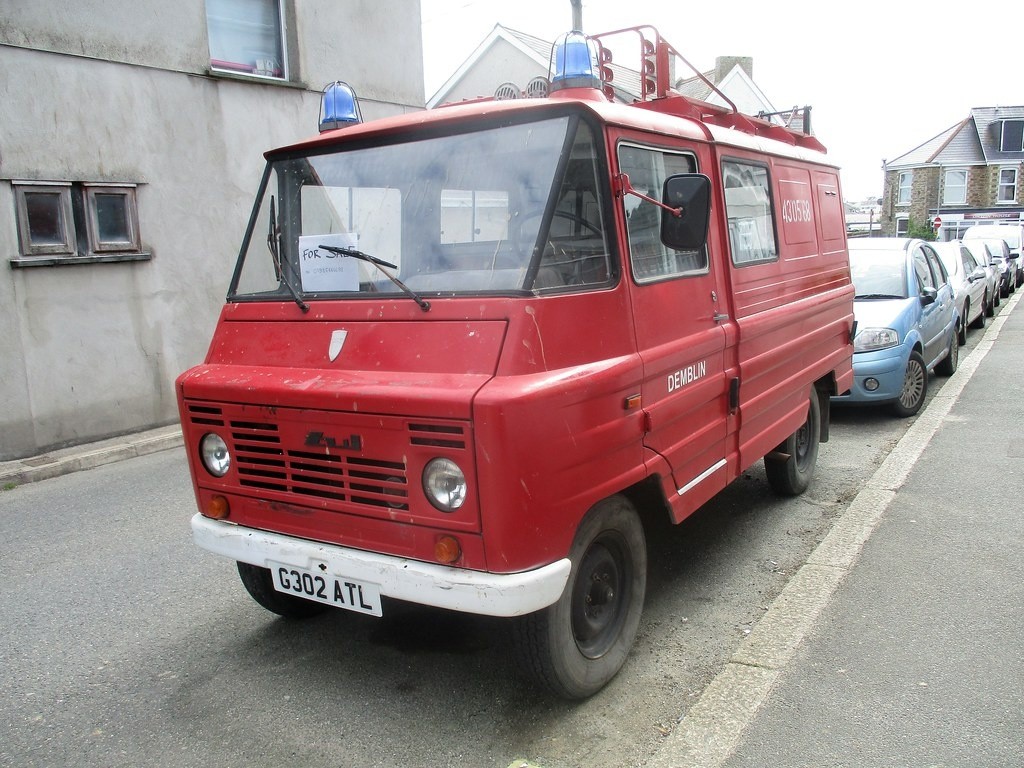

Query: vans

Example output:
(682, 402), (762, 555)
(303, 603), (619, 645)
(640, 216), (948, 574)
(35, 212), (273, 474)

(175, 25), (860, 705)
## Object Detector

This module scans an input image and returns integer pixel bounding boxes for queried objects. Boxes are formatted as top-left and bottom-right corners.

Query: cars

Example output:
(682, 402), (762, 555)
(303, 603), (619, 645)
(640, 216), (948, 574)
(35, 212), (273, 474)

(928, 223), (1024, 344)
(830, 239), (959, 421)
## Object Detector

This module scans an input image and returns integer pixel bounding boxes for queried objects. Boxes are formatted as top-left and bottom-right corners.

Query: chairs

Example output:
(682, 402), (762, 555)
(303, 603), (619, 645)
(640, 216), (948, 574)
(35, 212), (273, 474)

(878, 279), (903, 297)
